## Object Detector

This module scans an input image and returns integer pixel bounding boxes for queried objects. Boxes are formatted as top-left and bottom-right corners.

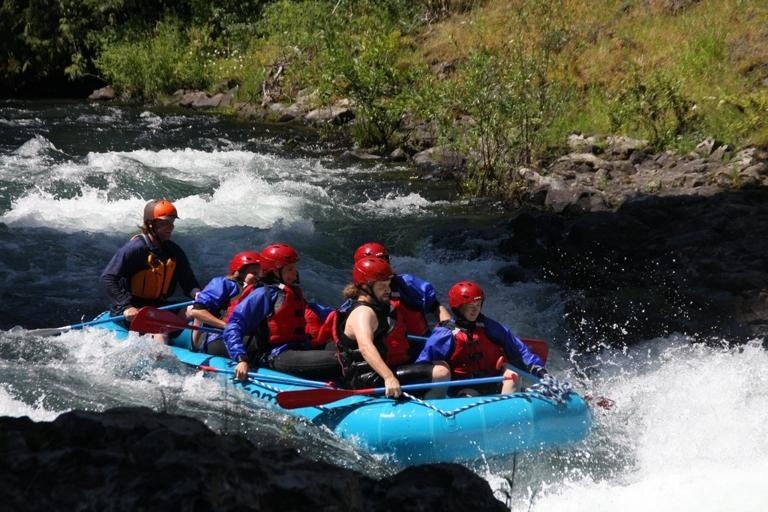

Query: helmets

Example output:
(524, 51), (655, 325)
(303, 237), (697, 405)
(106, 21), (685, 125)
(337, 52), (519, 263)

(145, 199), (181, 221)
(352, 242), (391, 288)
(230, 242), (299, 277)
(449, 282), (484, 308)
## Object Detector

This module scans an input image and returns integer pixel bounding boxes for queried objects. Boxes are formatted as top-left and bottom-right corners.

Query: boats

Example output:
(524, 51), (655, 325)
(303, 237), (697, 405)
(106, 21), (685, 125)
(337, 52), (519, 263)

(83, 306), (593, 472)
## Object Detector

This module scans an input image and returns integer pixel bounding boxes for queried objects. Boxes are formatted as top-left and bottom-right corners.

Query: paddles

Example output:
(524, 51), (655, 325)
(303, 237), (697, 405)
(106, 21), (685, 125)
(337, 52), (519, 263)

(130, 306), (226, 340)
(25, 300), (195, 338)
(158, 356), (336, 387)
(406, 338), (549, 366)
(277, 374), (518, 410)
(505, 364), (615, 409)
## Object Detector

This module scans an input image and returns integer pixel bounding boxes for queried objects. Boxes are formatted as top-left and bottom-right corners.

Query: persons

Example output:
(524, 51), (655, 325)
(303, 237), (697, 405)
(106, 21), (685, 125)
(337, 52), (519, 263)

(338, 256), (451, 399)
(99, 200), (201, 339)
(224, 243), (339, 381)
(339, 243), (451, 348)
(191, 251), (261, 358)
(414, 281), (553, 395)
